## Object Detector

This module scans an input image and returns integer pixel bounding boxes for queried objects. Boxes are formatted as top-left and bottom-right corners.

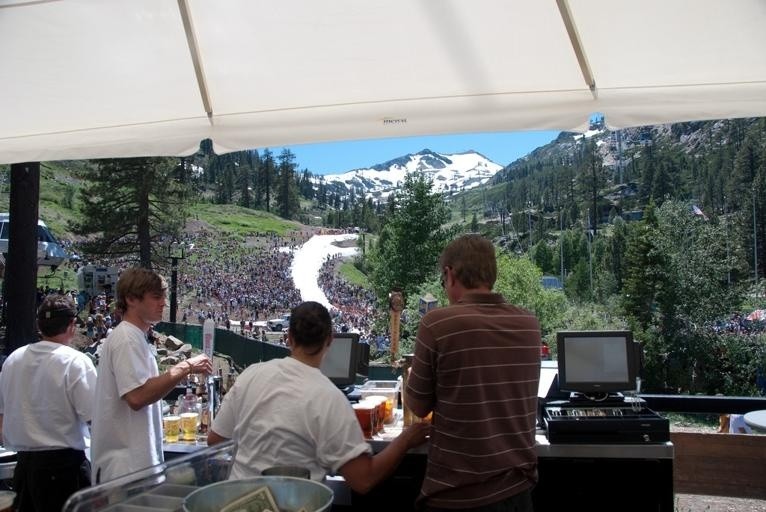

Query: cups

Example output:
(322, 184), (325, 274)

(352, 396), (387, 440)
(163, 413), (199, 443)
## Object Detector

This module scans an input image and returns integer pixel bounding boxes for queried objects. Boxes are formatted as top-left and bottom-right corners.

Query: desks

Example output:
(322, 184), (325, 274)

(744, 410), (766, 432)
(352, 409), (675, 512)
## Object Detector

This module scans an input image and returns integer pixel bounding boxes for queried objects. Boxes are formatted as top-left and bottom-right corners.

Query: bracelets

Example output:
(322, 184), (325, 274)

(186, 360), (193, 374)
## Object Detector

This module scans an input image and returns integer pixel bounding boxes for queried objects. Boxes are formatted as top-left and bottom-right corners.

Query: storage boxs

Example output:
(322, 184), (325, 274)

(359, 379), (401, 424)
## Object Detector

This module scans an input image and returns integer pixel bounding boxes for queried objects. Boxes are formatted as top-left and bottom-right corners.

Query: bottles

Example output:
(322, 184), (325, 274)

(178, 366), (236, 434)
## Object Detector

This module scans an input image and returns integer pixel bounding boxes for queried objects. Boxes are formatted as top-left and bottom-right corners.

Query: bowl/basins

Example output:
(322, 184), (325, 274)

(182, 476), (333, 512)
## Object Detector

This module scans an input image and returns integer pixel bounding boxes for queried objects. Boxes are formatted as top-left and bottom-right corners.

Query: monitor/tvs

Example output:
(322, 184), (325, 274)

(323, 333), (361, 385)
(556, 329), (635, 404)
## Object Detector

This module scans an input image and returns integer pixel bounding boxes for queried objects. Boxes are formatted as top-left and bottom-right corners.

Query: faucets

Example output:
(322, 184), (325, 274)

(206, 377), (221, 434)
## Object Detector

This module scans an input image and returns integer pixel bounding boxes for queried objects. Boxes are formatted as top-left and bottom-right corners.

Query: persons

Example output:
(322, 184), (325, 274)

(91, 268), (212, 486)
(35, 220), (316, 355)
(0, 298), (98, 512)
(407, 234), (543, 511)
(709, 309), (753, 337)
(318, 225), (369, 235)
(317, 253), (410, 355)
(207, 301), (435, 497)
